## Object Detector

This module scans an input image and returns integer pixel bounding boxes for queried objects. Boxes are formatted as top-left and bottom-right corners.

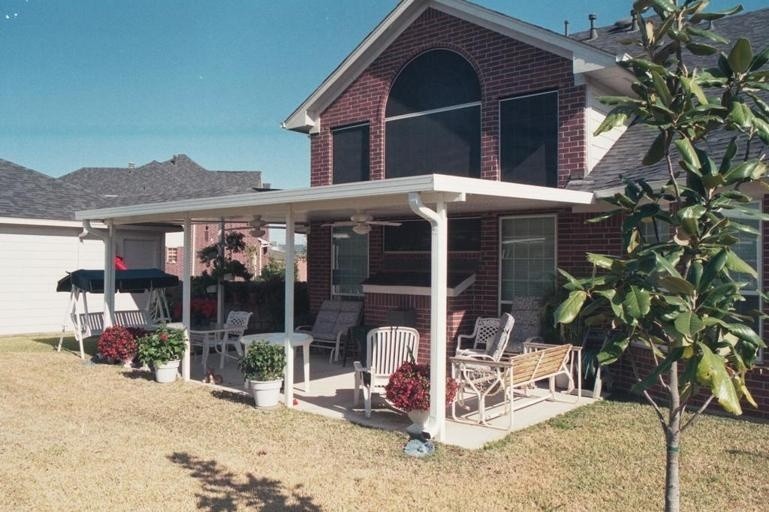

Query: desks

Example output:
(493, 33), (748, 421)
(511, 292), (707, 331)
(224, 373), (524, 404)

(190, 323), (241, 375)
(239, 333), (314, 392)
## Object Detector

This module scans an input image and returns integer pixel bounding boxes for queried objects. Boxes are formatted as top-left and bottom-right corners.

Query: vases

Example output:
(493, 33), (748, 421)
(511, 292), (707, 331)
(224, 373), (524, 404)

(153, 359), (181, 383)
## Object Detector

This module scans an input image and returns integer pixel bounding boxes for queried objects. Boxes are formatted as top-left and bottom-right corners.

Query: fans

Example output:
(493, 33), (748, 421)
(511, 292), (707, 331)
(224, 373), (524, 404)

(319, 209), (402, 235)
(224, 214), (287, 237)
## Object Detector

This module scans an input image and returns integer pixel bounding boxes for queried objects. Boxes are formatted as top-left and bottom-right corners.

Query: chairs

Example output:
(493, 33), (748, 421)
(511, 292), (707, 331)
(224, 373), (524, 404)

(353, 325), (420, 418)
(453, 312), (515, 397)
(220, 311), (253, 369)
(295, 299), (363, 364)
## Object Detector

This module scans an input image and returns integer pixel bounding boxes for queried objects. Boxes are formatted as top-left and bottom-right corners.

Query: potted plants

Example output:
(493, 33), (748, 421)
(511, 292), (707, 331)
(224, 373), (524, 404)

(212, 257), (254, 281)
(191, 268), (218, 294)
(224, 231), (246, 254)
(197, 244), (218, 264)
(98, 325), (138, 364)
(385, 361), (457, 435)
(237, 339), (288, 407)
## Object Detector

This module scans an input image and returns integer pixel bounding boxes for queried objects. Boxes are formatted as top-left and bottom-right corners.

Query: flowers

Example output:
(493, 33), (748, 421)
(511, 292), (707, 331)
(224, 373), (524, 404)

(136, 326), (189, 366)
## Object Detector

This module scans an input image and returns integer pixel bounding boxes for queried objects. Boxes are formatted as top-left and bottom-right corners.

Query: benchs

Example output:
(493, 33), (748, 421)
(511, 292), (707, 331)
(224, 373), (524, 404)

(503, 344), (574, 415)
(70, 310), (149, 341)
